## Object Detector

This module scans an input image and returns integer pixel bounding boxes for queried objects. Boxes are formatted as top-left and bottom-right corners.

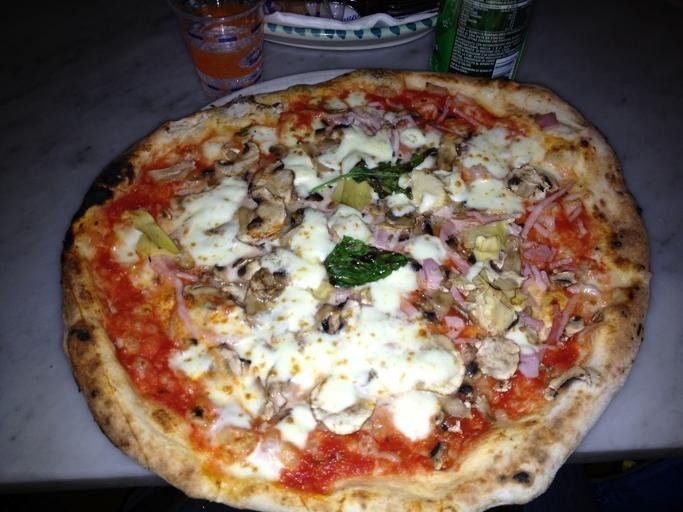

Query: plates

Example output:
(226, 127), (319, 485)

(263, 13), (438, 51)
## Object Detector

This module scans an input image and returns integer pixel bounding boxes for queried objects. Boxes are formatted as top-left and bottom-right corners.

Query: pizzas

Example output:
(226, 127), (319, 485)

(58, 67), (653, 509)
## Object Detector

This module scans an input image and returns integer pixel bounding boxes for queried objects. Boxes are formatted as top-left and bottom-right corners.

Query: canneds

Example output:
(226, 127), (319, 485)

(426, 0), (534, 81)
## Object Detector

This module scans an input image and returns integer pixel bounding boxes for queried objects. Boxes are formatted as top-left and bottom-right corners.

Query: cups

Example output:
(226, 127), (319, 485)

(170, 0), (268, 98)
